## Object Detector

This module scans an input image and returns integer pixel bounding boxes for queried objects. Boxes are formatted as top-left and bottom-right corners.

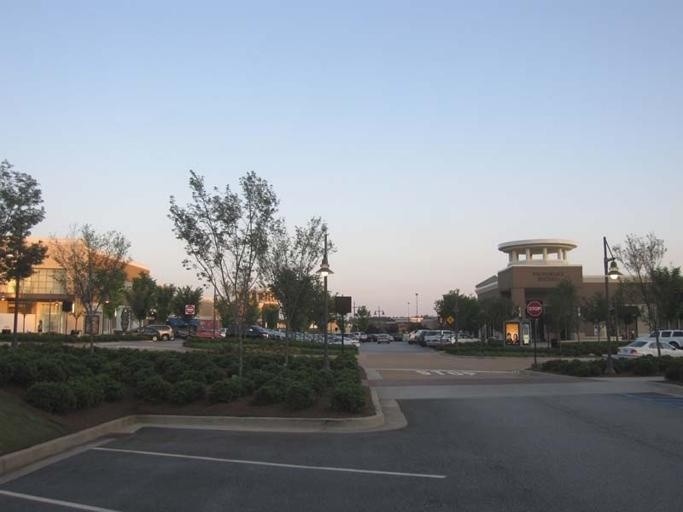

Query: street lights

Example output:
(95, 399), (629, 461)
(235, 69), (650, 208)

(414, 292), (419, 314)
(406, 301), (410, 314)
(601, 236), (622, 373)
(374, 306), (384, 331)
(316, 233), (331, 373)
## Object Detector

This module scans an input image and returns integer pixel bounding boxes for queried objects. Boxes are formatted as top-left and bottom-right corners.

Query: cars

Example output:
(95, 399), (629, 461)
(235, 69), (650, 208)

(616, 340), (682, 358)
(119, 318), (394, 348)
(406, 330), (481, 347)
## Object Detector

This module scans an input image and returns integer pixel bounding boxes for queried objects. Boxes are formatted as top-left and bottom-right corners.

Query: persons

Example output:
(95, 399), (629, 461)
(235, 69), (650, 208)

(38, 320), (42, 333)
(506, 332), (519, 345)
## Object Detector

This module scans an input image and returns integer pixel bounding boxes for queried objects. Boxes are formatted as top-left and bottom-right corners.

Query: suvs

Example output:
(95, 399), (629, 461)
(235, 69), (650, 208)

(636, 329), (682, 350)
(484, 336), (504, 346)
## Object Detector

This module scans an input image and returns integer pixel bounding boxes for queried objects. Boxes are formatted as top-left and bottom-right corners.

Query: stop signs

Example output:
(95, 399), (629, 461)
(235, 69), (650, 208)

(185, 305), (195, 315)
(527, 299), (543, 319)
